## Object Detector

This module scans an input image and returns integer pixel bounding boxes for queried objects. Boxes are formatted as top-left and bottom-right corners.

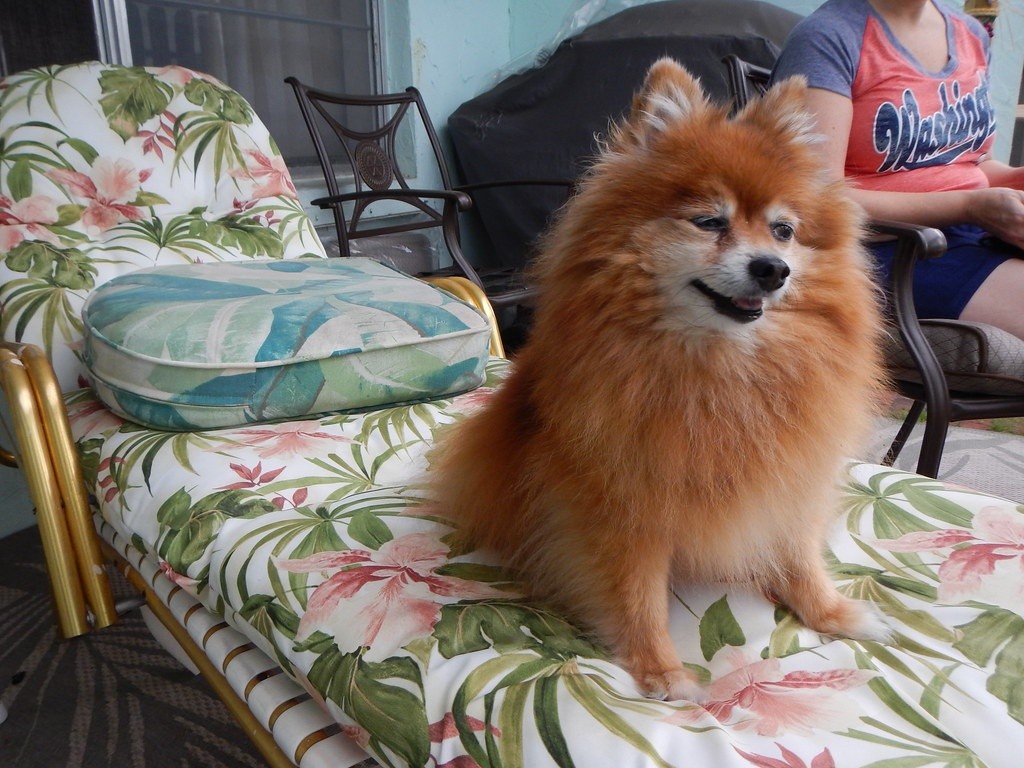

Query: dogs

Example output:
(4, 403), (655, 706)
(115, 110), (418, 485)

(422, 57), (895, 701)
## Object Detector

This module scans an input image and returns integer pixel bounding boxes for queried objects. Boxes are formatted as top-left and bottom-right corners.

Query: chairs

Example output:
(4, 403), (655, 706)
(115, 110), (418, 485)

(0, 60), (1024, 768)
(283, 75), (542, 342)
(719, 53), (1024, 480)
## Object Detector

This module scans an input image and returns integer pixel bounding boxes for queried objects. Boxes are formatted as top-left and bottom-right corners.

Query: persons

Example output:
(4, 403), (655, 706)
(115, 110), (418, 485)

(768, 1), (1023, 345)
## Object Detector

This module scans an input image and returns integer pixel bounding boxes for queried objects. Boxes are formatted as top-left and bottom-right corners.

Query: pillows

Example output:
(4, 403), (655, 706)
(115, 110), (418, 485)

(81, 254), (491, 432)
